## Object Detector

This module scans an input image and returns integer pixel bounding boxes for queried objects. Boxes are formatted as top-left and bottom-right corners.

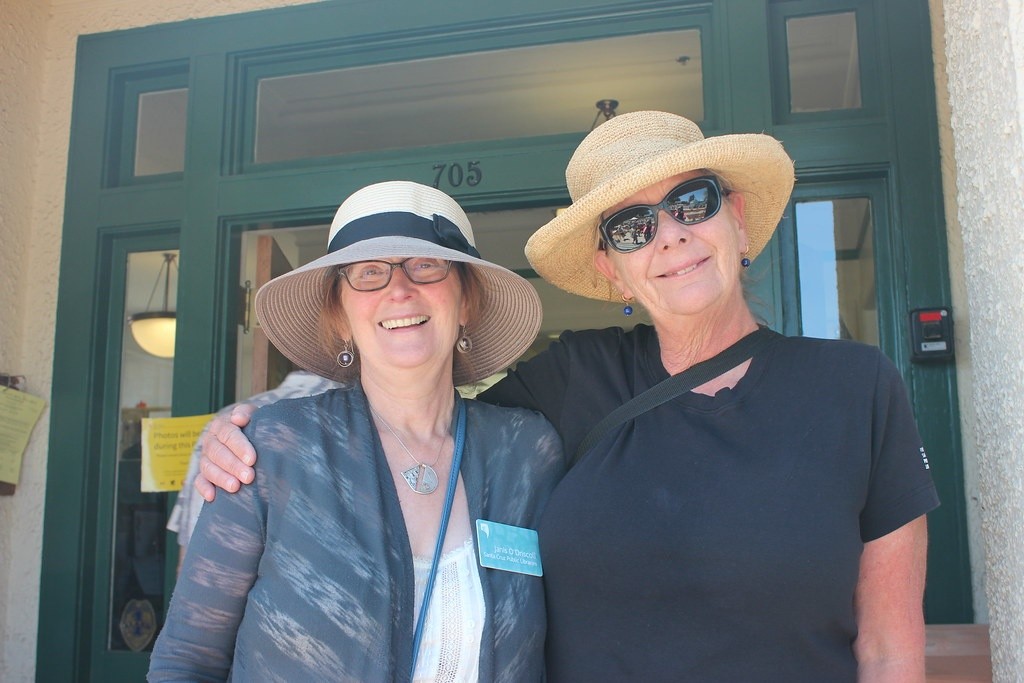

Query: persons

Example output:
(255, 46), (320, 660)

(612, 218), (653, 244)
(193, 111), (940, 683)
(670, 206), (686, 221)
(144, 180), (563, 681)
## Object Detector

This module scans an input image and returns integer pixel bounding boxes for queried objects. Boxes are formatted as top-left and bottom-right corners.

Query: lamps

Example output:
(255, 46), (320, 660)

(127, 254), (177, 358)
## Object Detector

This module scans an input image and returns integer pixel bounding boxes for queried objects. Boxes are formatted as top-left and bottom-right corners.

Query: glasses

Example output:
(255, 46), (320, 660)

(598, 172), (730, 254)
(334, 256), (454, 292)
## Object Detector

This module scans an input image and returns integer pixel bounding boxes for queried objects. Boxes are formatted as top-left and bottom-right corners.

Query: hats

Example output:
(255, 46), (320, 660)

(524, 110), (794, 304)
(253, 181), (543, 386)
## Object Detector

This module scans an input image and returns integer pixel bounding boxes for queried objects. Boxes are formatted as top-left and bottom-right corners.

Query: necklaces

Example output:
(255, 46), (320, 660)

(367, 404), (457, 494)
